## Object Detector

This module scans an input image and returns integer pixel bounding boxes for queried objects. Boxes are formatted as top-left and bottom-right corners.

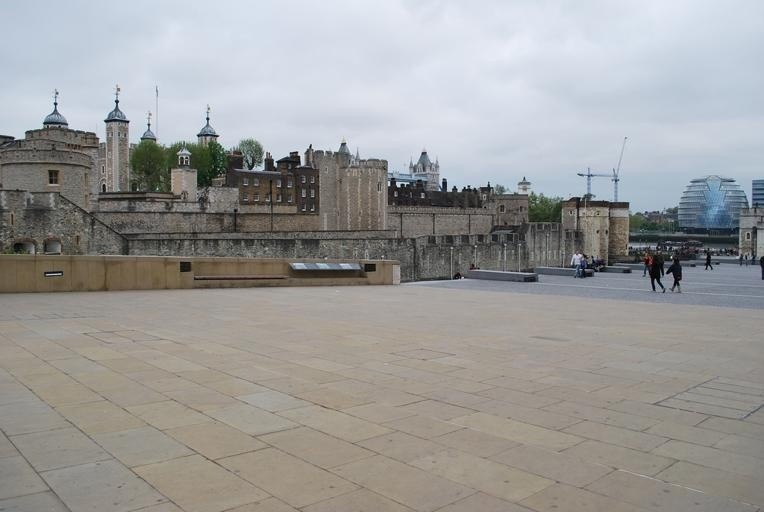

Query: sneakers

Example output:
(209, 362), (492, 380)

(705, 268), (713, 270)
(642, 274), (682, 293)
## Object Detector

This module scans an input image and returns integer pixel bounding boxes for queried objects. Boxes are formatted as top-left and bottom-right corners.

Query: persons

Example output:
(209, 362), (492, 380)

(629, 245), (764, 293)
(570, 250), (596, 278)
(470, 264), (475, 270)
(453, 273), (461, 280)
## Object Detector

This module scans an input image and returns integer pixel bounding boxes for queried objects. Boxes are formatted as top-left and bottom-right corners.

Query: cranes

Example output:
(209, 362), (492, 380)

(576, 167), (619, 200)
(612, 134), (631, 202)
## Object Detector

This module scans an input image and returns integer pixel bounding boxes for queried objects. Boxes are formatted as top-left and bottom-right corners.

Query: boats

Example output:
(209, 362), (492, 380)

(664, 239), (704, 246)
